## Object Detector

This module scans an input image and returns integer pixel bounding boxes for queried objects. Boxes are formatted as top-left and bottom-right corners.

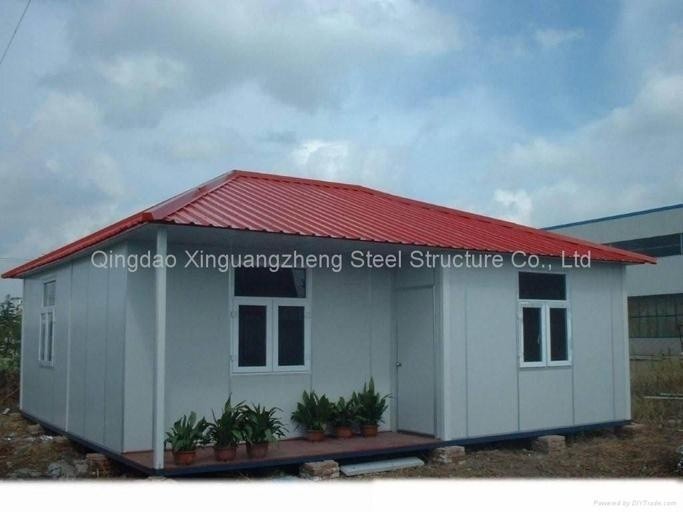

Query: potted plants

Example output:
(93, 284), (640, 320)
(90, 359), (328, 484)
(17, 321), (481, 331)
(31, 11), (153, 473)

(163, 375), (394, 466)
